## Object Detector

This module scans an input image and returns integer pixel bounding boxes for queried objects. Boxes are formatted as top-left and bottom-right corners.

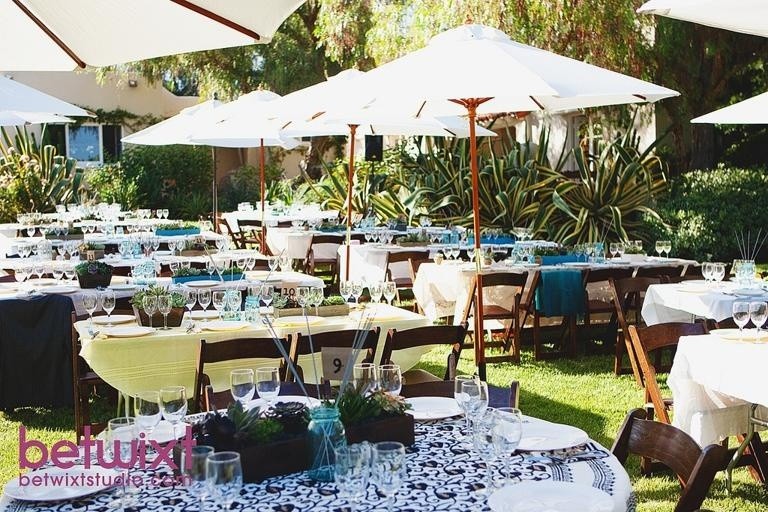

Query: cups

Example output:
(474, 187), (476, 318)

(733, 259), (760, 290)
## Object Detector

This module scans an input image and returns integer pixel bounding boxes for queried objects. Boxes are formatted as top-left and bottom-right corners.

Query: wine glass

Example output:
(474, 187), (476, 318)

(406, 215), (676, 267)
(732, 300), (768, 341)
(108, 385), (190, 509)
(453, 373), (523, 496)
(14, 198), (396, 331)
(180, 445), (245, 511)
(228, 366), (282, 408)
(333, 440), (406, 512)
(352, 362), (405, 401)
(702, 259), (726, 289)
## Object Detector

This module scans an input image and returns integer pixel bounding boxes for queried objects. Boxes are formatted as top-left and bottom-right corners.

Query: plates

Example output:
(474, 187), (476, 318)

(406, 394), (467, 421)
(99, 325), (156, 338)
(245, 394), (321, 412)
(4, 462), (116, 501)
(512, 417), (588, 451)
(487, 473), (615, 512)
(710, 328), (768, 344)
(676, 279), (768, 297)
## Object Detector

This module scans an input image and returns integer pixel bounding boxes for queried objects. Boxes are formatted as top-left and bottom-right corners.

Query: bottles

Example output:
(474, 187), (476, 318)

(305, 403), (350, 484)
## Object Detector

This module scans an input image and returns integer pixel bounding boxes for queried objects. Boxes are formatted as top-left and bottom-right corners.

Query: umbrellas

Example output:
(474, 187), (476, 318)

(119, 92), (286, 234)
(636, 0), (768, 41)
(251, 65), (498, 280)
(296, 15), (683, 365)
(688, 90), (768, 128)
(185, 86), (299, 252)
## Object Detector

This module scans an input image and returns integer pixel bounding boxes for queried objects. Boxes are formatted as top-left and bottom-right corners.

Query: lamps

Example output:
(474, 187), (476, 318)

(129, 79), (137, 88)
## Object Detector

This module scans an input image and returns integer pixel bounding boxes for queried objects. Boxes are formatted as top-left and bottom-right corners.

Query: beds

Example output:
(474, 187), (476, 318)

(0, 210), (768, 512)
